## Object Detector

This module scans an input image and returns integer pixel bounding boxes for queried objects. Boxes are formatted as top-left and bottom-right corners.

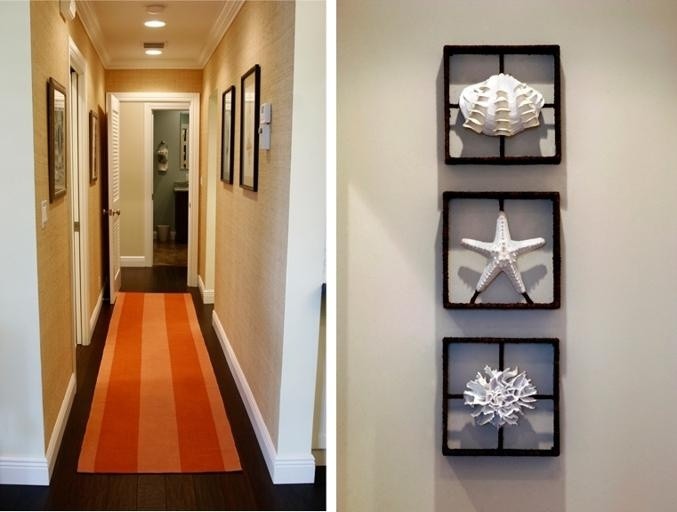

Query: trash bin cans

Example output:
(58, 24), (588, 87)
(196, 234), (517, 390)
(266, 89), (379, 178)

(157, 224), (169, 242)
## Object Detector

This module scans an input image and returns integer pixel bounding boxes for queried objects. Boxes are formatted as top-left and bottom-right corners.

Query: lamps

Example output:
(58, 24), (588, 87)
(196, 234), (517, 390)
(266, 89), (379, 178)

(144, 6), (167, 27)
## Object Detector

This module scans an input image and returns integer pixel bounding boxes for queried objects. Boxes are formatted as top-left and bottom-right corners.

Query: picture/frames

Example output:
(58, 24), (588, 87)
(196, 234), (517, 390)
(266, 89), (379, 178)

(221, 85), (236, 185)
(443, 191), (561, 309)
(240, 64), (261, 191)
(442, 337), (560, 457)
(49, 77), (67, 203)
(443, 45), (561, 165)
(90, 109), (97, 185)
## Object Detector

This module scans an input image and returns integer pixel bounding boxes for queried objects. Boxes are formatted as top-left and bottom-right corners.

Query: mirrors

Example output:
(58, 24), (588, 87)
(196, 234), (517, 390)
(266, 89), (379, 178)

(179, 111), (189, 169)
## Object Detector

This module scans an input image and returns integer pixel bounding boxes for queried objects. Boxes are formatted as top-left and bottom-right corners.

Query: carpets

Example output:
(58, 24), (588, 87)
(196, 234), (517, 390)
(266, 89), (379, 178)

(76, 292), (244, 473)
